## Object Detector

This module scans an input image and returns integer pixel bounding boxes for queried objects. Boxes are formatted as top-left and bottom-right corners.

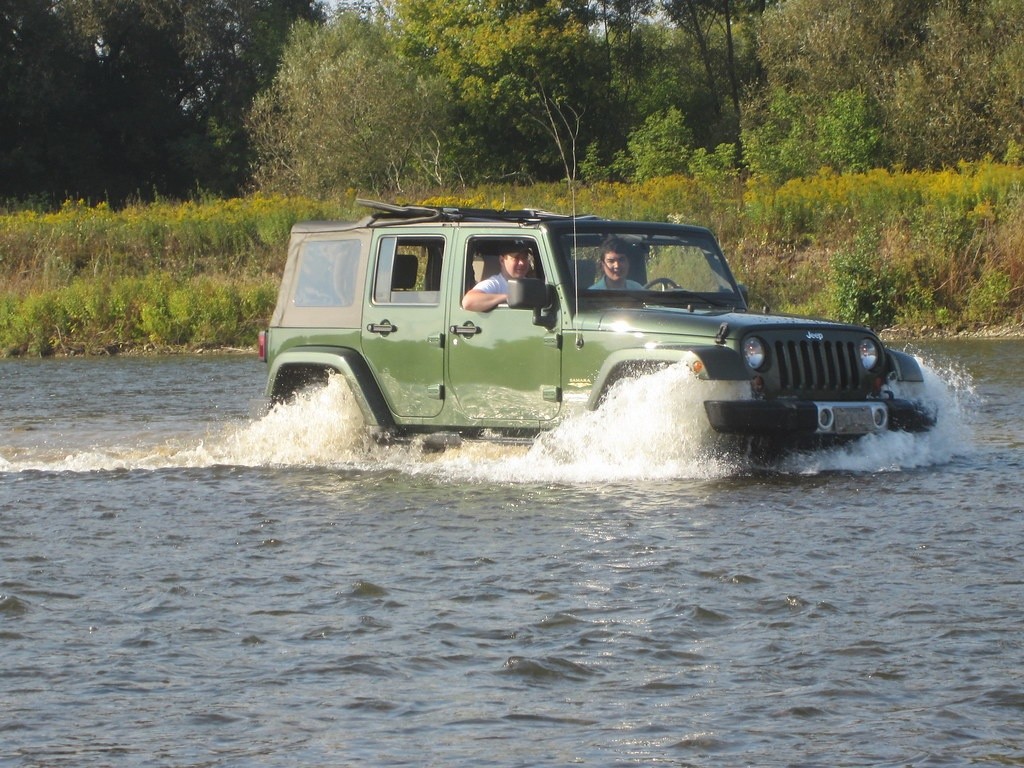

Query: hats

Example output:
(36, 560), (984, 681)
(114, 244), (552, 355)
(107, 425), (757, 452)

(498, 239), (534, 256)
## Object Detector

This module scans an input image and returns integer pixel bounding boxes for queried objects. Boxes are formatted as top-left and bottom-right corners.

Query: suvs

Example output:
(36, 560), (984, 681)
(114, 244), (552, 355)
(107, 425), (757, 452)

(259, 193), (936, 447)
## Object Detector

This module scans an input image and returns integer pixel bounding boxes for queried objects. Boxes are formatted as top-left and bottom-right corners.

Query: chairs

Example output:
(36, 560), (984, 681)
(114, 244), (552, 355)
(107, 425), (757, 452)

(571, 260), (597, 291)
(391, 255), (417, 292)
(472, 255), (501, 283)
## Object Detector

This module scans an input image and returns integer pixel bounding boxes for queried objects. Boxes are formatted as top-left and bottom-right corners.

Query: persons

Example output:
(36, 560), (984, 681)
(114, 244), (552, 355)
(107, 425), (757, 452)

(459, 239), (531, 312)
(588, 235), (644, 291)
